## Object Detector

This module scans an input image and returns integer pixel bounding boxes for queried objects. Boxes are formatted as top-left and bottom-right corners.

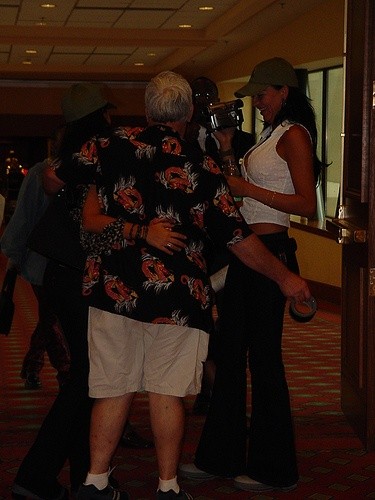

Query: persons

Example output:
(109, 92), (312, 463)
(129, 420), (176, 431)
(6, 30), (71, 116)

(1, 57), (333, 500)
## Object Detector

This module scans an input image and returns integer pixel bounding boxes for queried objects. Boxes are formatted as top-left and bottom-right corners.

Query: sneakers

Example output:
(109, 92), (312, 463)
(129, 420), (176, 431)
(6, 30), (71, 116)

(232, 471), (299, 493)
(156, 486), (195, 500)
(80, 482), (126, 500)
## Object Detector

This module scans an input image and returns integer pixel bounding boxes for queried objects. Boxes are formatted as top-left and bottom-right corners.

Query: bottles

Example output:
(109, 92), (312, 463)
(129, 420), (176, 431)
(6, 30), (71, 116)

(223, 159), (243, 207)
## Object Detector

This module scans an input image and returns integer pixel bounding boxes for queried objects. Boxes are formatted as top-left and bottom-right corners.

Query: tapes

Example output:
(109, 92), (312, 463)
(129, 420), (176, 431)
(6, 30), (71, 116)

(289, 294), (318, 323)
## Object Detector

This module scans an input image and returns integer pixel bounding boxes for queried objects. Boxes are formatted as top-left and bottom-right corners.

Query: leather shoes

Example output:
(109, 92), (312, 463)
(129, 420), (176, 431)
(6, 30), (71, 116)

(18, 370), (44, 389)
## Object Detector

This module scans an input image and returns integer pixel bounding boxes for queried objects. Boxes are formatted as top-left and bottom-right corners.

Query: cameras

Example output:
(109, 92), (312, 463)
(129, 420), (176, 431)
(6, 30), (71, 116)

(202, 99), (244, 133)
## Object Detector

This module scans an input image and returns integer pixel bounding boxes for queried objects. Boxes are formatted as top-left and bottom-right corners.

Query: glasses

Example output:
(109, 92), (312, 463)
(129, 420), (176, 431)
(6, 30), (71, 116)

(192, 91), (214, 101)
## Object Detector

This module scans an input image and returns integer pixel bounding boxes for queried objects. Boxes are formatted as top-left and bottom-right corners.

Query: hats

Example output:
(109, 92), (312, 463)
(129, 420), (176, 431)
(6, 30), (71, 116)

(233, 57), (294, 98)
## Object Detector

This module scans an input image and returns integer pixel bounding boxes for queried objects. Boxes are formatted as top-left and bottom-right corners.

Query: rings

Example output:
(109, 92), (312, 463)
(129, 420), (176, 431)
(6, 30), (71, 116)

(166, 242), (171, 246)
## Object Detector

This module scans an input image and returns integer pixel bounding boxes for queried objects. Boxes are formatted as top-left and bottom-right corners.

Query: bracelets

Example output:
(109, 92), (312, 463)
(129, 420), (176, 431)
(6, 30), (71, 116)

(142, 222), (148, 239)
(263, 190), (276, 206)
(136, 222), (143, 239)
(129, 222), (138, 240)
